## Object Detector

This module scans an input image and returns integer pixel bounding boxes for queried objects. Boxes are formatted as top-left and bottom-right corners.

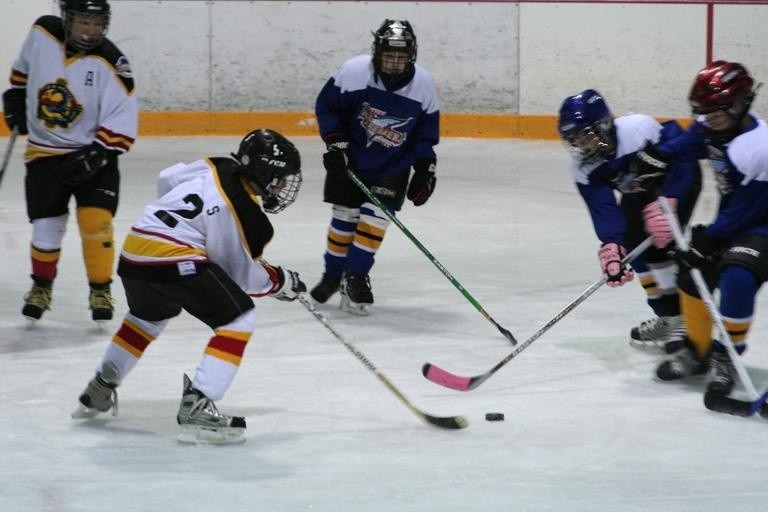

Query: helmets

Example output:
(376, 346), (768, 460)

(371, 19), (417, 80)
(58, 1), (110, 52)
(689, 60), (756, 129)
(235, 129), (302, 214)
(558, 89), (615, 165)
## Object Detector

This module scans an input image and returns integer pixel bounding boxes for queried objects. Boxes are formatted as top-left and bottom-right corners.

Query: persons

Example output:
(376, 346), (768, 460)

(1, 0), (140, 321)
(70, 128), (307, 429)
(634, 59), (768, 397)
(307, 17), (442, 304)
(556, 88), (704, 342)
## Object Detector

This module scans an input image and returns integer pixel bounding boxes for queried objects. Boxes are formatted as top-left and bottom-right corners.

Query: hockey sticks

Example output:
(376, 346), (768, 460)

(705, 388), (768, 416)
(423, 235), (652, 391)
(346, 169), (518, 345)
(655, 186), (767, 422)
(298, 294), (470, 429)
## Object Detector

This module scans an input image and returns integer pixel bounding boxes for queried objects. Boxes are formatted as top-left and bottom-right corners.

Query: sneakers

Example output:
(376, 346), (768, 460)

(310, 273), (341, 305)
(345, 272), (374, 305)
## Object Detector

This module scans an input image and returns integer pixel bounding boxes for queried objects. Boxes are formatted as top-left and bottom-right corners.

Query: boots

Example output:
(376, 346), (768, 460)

(22, 275), (56, 321)
(89, 279), (113, 320)
(631, 317), (745, 395)
(78, 359), (121, 412)
(177, 374), (247, 429)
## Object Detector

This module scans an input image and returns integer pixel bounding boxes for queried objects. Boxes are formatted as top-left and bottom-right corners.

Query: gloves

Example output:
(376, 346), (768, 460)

(612, 140), (671, 197)
(406, 155), (437, 206)
(322, 132), (356, 181)
(267, 266), (307, 302)
(641, 197), (680, 251)
(2, 88), (29, 136)
(599, 243), (635, 287)
(55, 141), (117, 185)
